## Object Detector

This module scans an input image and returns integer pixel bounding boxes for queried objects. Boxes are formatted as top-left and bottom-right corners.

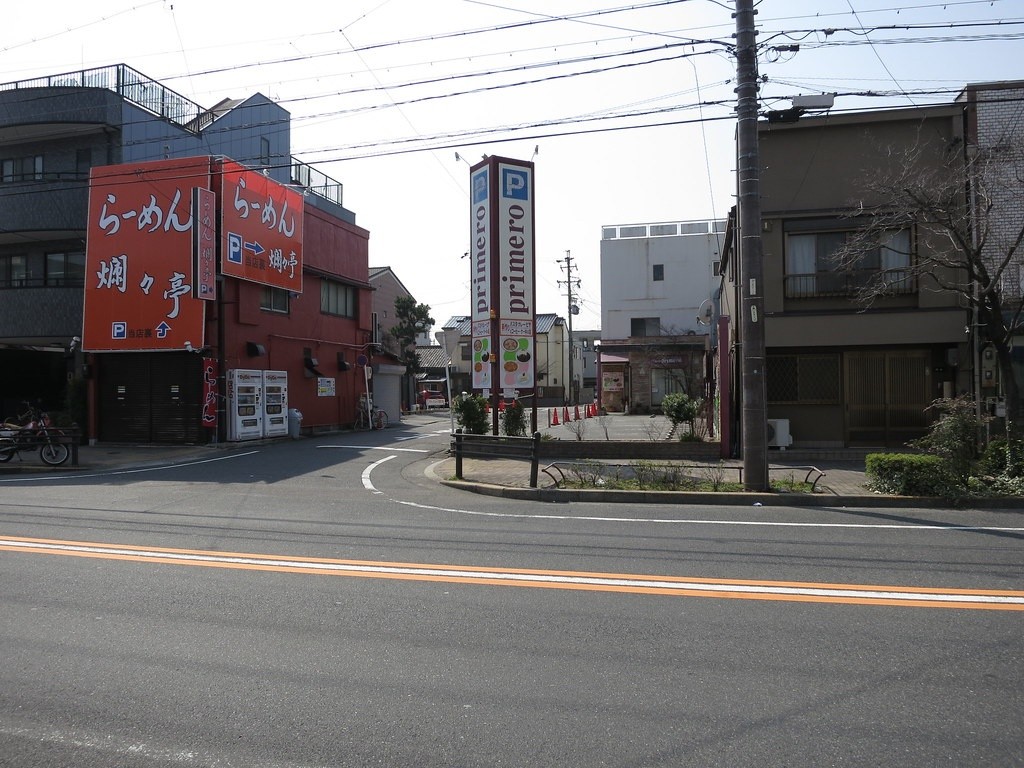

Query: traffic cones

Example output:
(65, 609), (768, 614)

(552, 402), (598, 426)
(374, 411), (385, 428)
(485, 398), (517, 412)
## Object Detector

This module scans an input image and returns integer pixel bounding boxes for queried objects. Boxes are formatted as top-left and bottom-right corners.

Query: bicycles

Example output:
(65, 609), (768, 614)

(353, 392), (388, 431)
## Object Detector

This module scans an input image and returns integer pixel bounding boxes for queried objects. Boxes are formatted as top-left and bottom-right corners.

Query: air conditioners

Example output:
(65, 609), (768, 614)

(768, 419), (792, 451)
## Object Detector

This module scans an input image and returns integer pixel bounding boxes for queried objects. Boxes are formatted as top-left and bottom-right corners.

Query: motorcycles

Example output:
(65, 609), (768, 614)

(0, 399), (71, 466)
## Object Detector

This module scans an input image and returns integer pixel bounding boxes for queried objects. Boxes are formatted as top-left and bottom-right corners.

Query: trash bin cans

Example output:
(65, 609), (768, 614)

(288, 408), (303, 439)
